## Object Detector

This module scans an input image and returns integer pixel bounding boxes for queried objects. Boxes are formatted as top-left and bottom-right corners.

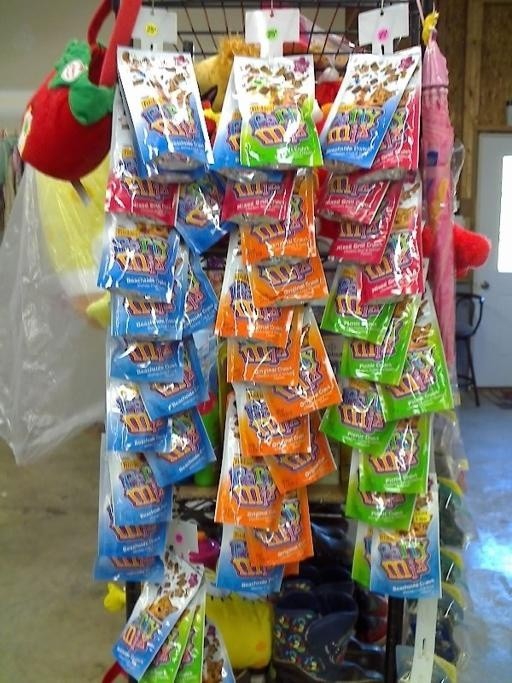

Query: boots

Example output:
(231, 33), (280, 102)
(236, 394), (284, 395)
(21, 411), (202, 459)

(268, 588), (385, 683)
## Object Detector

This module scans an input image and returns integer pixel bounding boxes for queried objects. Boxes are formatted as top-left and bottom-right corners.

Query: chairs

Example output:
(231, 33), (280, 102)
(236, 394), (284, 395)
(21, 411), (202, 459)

(456, 292), (485, 408)
